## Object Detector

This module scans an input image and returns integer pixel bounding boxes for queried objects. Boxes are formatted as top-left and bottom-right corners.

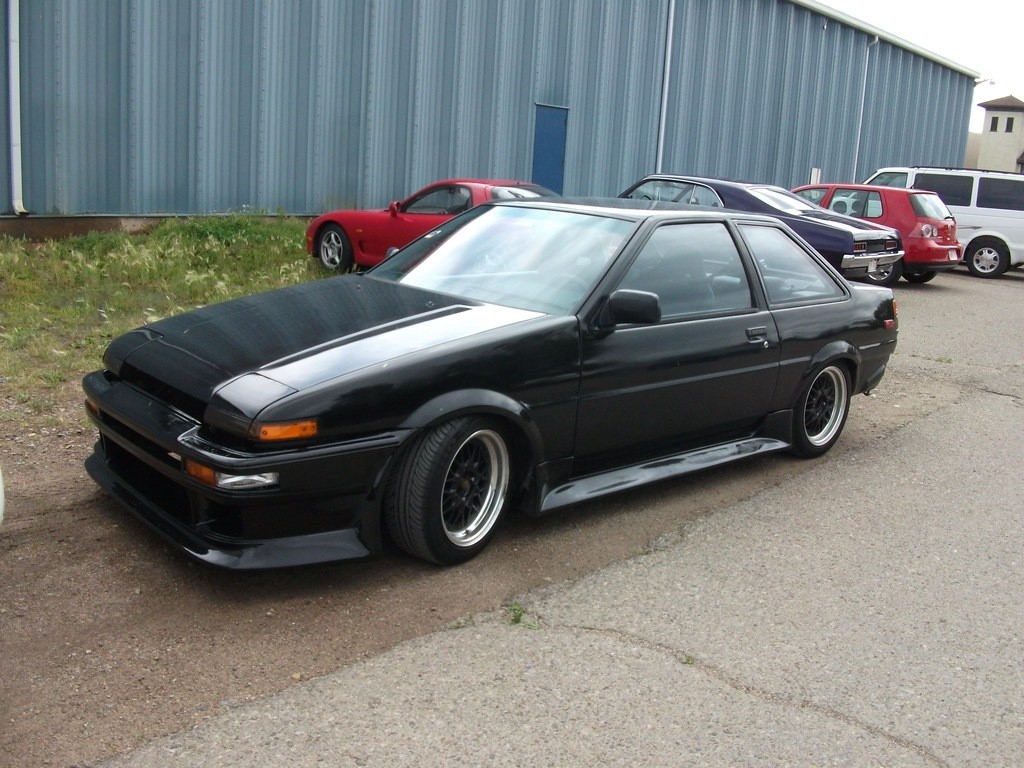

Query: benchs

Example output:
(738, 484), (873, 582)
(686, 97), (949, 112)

(708, 272), (823, 306)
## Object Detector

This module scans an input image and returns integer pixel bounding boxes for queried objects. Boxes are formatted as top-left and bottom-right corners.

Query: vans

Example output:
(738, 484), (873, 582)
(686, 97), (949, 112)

(857, 164), (1024, 280)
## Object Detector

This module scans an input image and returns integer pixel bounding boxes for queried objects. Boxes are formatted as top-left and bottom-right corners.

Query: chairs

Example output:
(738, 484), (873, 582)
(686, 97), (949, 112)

(626, 246), (717, 311)
(849, 200), (864, 217)
(832, 201), (847, 214)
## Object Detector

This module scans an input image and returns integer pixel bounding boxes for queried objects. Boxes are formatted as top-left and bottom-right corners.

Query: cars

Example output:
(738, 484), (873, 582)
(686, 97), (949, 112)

(304, 180), (561, 274)
(616, 174), (905, 280)
(82, 199), (898, 573)
(790, 186), (963, 287)
